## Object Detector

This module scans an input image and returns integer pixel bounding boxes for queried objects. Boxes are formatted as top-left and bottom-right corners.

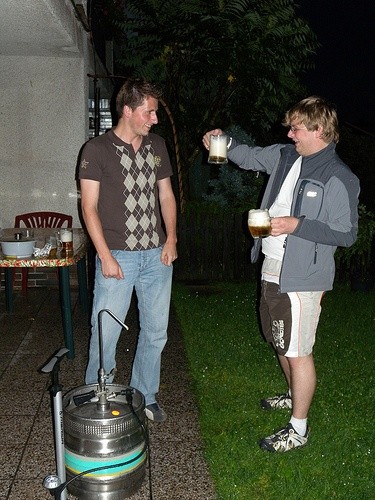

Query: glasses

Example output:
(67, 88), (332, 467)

(289, 125), (309, 133)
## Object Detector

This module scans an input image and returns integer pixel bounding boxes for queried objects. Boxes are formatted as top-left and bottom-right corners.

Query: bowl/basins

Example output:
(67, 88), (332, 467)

(0, 241), (35, 256)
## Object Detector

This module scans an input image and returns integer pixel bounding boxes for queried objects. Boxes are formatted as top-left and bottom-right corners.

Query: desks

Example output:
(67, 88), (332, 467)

(0, 228), (90, 358)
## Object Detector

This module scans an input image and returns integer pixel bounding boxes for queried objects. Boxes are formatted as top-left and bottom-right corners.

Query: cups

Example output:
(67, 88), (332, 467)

(45, 236), (62, 254)
(207, 134), (227, 164)
(59, 228), (73, 251)
(248, 209), (274, 239)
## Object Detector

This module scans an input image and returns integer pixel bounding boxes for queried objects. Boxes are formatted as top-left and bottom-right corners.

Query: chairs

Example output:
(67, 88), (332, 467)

(12, 211), (73, 295)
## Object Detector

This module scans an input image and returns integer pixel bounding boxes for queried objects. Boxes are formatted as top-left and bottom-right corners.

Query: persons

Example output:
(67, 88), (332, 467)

(77, 80), (178, 423)
(202, 96), (360, 451)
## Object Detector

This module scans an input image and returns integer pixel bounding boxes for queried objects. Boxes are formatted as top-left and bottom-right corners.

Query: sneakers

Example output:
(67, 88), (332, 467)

(144, 402), (167, 423)
(258, 422), (311, 452)
(260, 393), (295, 410)
